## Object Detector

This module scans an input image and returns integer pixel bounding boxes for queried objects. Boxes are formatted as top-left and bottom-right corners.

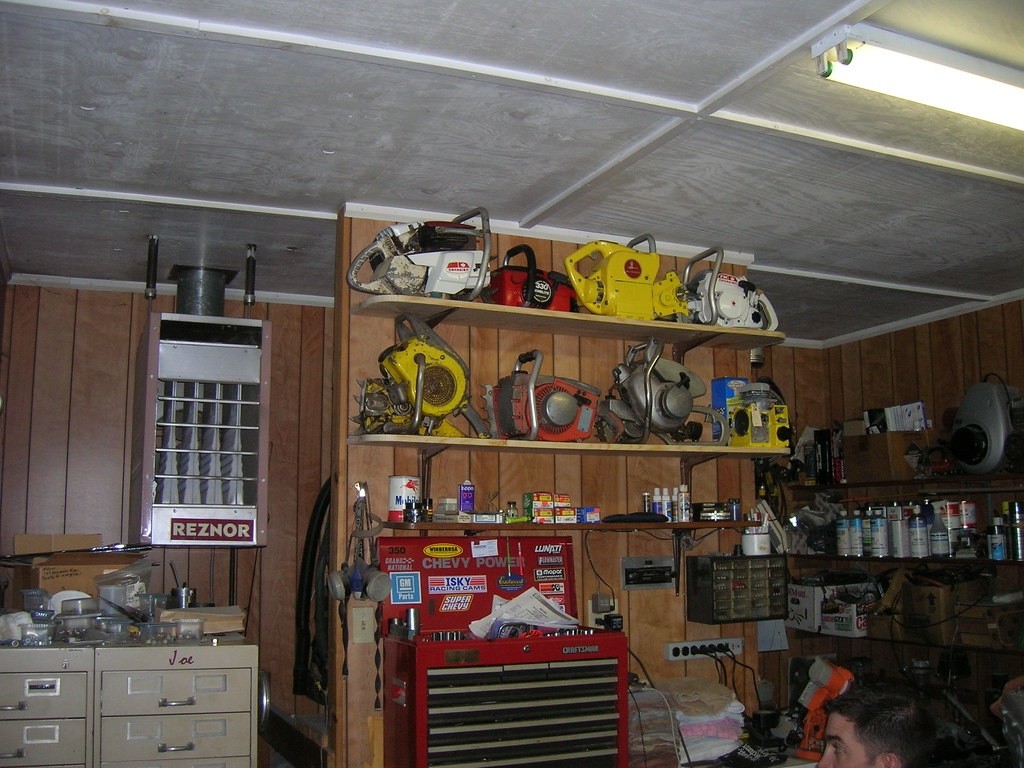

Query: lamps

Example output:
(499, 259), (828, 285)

(811, 24), (1024, 133)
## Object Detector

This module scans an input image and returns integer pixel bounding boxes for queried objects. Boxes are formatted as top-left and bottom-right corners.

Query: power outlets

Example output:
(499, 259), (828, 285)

(585, 599), (619, 630)
(664, 637), (742, 661)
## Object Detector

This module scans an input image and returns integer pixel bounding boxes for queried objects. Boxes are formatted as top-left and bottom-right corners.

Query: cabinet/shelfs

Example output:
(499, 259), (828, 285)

(332, 204), (793, 768)
(0, 633), (259, 768)
(785, 482), (1024, 768)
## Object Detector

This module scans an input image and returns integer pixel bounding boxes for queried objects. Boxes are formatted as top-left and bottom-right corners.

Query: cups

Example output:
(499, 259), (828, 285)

(742, 533), (770, 555)
(171, 586), (196, 608)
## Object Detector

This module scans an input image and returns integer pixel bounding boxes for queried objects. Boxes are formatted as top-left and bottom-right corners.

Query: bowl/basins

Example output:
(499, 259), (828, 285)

(172, 618), (208, 643)
(18, 584), (133, 646)
(135, 623), (178, 645)
(134, 593), (168, 619)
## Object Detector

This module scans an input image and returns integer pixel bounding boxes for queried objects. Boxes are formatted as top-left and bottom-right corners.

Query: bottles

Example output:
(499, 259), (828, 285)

(862, 498), (949, 558)
(835, 510), (862, 556)
(652, 487), (672, 523)
(986, 500), (1024, 561)
(672, 484), (690, 523)
(506, 501), (518, 516)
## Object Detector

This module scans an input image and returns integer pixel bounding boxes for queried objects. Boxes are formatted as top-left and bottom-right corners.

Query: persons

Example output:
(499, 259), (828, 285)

(817, 682), (940, 768)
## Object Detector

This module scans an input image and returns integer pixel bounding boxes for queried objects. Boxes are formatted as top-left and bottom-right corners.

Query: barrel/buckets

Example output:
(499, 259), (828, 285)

(387, 475), (421, 523)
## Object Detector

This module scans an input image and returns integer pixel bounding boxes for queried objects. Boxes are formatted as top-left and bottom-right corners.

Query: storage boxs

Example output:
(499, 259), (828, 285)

(844, 428), (936, 484)
(787, 581), (877, 634)
(821, 599), (884, 639)
(899, 575), (989, 649)
(956, 599), (1024, 651)
(867, 612), (903, 641)
(0, 534), (150, 614)
